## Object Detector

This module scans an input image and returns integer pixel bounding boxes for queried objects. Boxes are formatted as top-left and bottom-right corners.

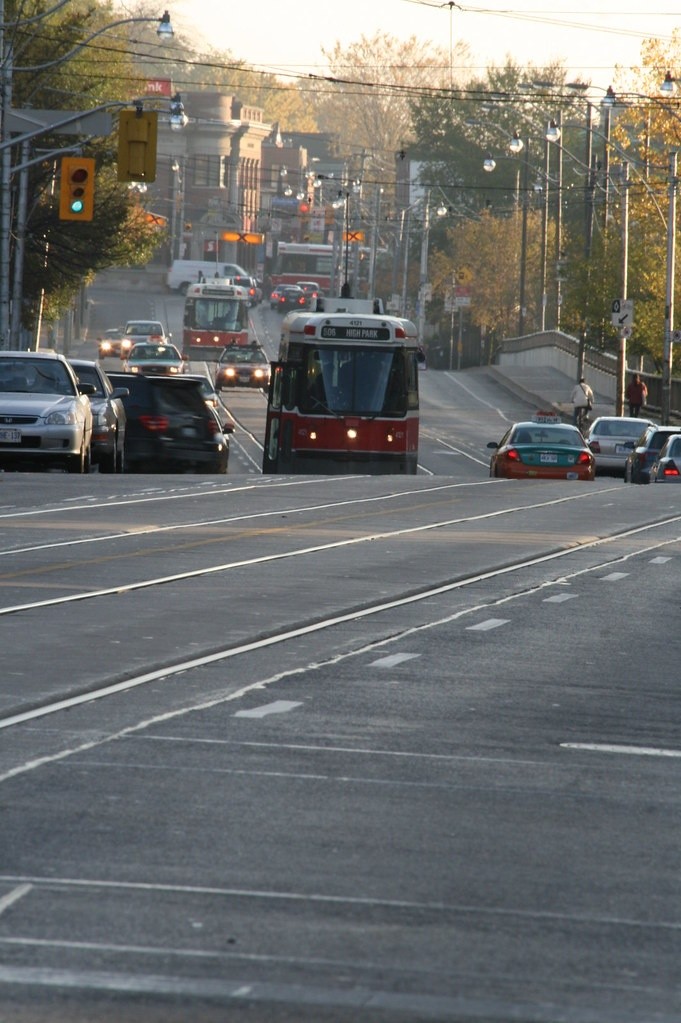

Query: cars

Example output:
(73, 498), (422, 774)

(0, 351), (97, 474)
(487, 415), (680, 485)
(215, 340), (271, 391)
(228, 275), (324, 314)
(173, 373), (219, 411)
(68, 359), (131, 474)
(97, 321), (189, 375)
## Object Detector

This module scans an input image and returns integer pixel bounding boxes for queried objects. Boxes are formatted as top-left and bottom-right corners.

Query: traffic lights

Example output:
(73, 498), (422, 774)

(60, 156), (96, 221)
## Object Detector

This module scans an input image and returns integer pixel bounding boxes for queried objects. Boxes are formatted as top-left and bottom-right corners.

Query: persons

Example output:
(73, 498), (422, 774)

(625, 374), (649, 417)
(571, 380), (595, 426)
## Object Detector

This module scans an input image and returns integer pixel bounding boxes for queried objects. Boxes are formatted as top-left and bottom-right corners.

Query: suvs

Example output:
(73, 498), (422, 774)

(105, 370), (227, 476)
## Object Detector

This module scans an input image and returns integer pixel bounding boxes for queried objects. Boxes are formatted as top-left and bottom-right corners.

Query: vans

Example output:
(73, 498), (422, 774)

(167, 259), (256, 295)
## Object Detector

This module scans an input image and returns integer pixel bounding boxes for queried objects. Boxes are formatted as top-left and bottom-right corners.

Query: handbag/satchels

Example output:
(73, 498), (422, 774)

(588, 399), (592, 410)
(640, 390), (647, 406)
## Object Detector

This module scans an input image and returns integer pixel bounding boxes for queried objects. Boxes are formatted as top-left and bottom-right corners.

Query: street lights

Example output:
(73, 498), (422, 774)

(484, 152), (598, 385)
(10, 90), (185, 354)
(508, 130), (630, 416)
(566, 82), (652, 176)
(0, 9), (175, 352)
(463, 80), (611, 351)
(546, 118), (678, 426)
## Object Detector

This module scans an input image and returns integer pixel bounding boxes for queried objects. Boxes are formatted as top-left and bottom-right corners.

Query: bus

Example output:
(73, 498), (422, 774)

(269, 241), (388, 298)
(183, 277), (249, 362)
(262, 298), (425, 474)
